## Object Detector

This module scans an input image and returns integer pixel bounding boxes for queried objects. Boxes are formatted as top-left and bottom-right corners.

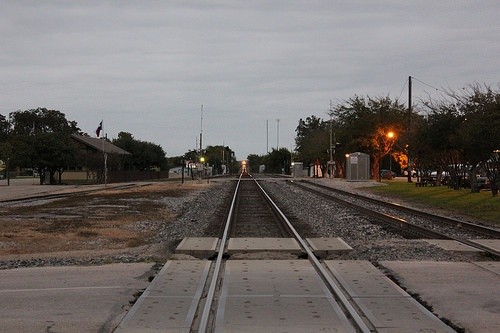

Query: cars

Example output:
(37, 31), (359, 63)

(429, 164), (498, 191)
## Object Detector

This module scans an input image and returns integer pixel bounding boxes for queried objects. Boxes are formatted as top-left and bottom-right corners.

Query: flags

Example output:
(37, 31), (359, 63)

(96, 121), (102, 137)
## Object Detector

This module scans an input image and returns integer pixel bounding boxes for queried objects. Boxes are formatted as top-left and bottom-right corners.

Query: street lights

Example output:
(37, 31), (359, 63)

(388, 133), (393, 173)
